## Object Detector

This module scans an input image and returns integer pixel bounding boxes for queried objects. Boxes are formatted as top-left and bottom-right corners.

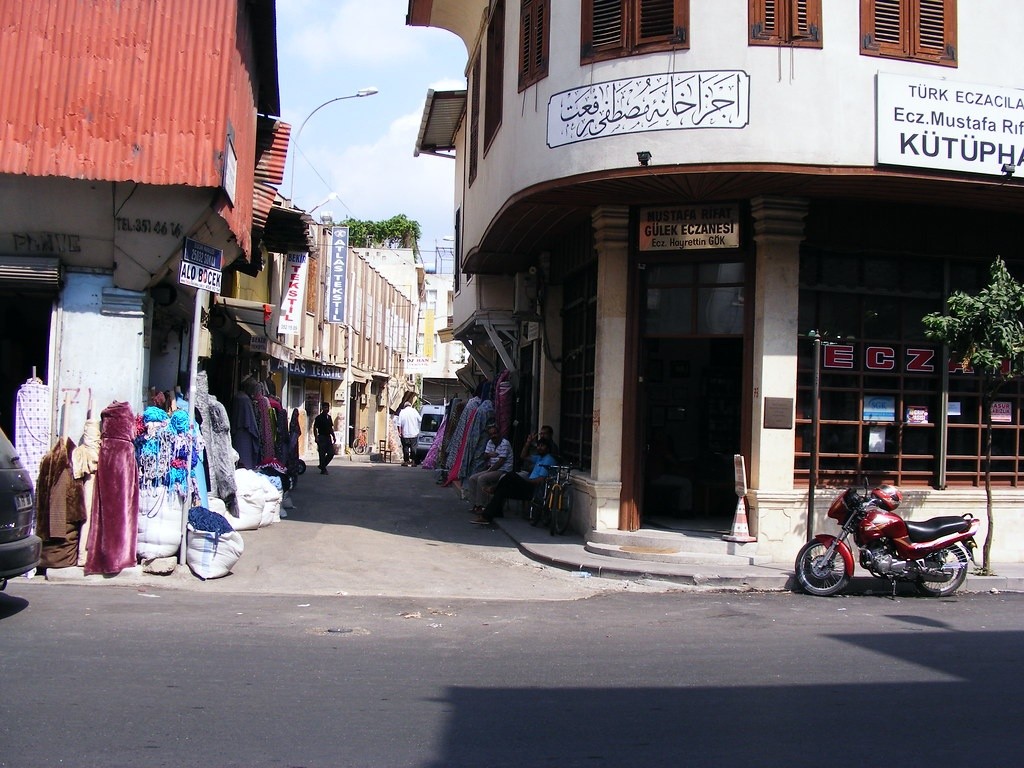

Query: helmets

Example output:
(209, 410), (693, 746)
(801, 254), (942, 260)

(872, 484), (903, 511)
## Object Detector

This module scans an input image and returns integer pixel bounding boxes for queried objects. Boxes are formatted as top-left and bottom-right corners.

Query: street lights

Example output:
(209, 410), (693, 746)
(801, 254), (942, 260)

(290, 85), (379, 210)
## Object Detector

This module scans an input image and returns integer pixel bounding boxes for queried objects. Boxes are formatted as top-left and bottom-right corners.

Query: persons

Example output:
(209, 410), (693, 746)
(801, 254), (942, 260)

(224, 377), (261, 469)
(468, 425), (513, 514)
(397, 400), (422, 466)
(483, 425), (558, 493)
(313, 403), (337, 476)
(468, 431), (557, 525)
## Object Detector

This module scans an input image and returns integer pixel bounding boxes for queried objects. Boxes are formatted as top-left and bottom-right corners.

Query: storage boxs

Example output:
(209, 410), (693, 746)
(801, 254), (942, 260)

(905, 405), (929, 423)
(863, 396), (895, 422)
(867, 426), (886, 453)
(948, 402), (961, 416)
(989, 401), (1012, 422)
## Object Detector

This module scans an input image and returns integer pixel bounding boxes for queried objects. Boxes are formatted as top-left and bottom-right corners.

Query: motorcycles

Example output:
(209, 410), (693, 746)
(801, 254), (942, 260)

(794, 474), (978, 601)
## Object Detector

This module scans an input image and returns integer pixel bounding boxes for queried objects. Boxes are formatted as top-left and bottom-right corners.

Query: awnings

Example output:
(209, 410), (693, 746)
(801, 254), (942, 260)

(265, 200), (316, 261)
(413, 90), (467, 154)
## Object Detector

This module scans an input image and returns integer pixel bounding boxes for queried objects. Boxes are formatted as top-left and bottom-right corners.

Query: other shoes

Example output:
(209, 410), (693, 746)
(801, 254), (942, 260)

(401, 463), (408, 467)
(317, 465), (322, 470)
(321, 470), (328, 475)
(411, 464), (417, 467)
(469, 515), (489, 524)
(483, 486), (495, 493)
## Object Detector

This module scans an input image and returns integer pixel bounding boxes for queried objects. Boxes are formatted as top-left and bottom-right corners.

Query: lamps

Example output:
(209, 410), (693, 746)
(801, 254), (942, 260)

(814, 329), (821, 339)
(1001, 164), (1017, 180)
(637, 151), (652, 166)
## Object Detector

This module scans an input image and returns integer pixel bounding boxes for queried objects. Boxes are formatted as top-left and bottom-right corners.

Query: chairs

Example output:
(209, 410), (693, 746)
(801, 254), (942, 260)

(380, 440), (392, 462)
(505, 481), (525, 516)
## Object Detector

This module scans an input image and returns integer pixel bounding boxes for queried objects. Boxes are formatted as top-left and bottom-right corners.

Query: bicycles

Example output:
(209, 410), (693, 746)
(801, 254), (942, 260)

(352, 426), (371, 455)
(529, 462), (577, 537)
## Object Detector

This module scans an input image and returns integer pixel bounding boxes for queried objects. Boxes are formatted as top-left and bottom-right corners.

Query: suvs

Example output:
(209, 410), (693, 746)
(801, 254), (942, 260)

(0, 426), (42, 591)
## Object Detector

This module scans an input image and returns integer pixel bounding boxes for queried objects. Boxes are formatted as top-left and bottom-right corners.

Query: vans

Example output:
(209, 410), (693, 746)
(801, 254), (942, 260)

(413, 405), (447, 465)
